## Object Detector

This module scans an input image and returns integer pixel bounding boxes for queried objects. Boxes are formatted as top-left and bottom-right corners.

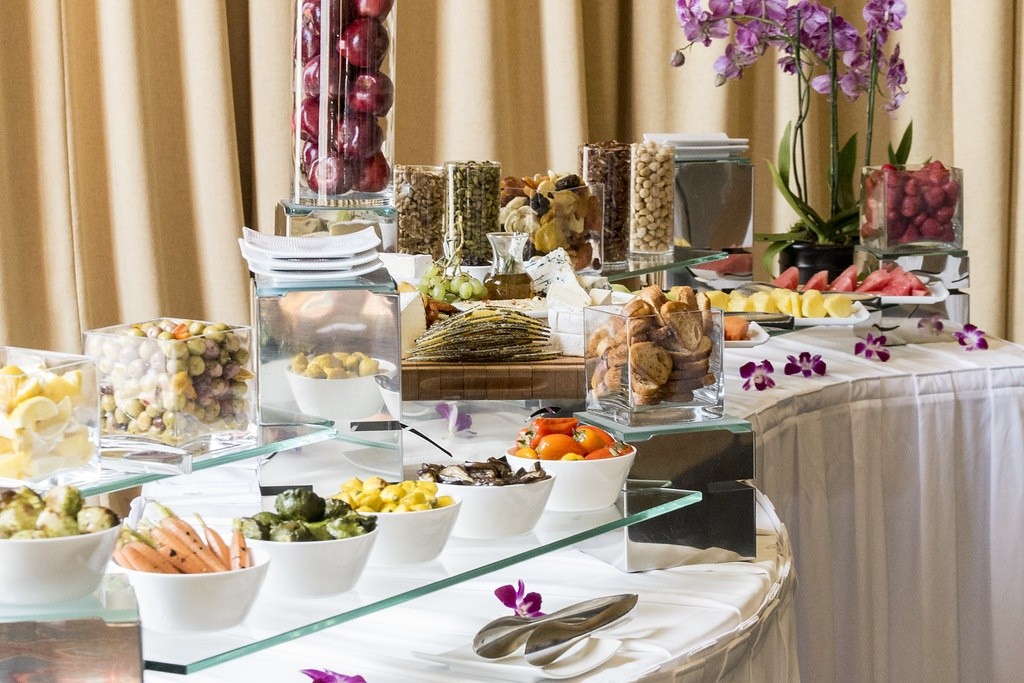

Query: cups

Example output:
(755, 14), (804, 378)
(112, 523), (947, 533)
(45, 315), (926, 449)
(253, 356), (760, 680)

(393, 164), (445, 261)
(579, 147), (631, 268)
(443, 159), (502, 267)
(627, 143), (677, 262)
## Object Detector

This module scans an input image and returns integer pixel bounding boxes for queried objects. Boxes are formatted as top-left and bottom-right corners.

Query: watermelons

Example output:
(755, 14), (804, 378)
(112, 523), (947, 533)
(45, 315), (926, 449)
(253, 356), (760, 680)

(769, 264), (930, 297)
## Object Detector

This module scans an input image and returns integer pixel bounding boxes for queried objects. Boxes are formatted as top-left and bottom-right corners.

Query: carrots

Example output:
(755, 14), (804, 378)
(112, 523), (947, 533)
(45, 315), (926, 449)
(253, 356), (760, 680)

(111, 511), (247, 574)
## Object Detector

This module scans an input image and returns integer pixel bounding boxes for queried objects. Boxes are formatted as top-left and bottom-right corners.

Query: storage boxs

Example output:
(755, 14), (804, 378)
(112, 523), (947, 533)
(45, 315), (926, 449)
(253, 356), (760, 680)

(0, 344), (101, 492)
(250, 249), (400, 427)
(631, 142), (674, 263)
(393, 164), (446, 263)
(583, 303), (725, 427)
(577, 144), (631, 271)
(288, 0), (398, 207)
(859, 162), (964, 255)
(82, 317), (259, 454)
(641, 131), (755, 248)
(446, 162), (501, 286)
(501, 177), (604, 280)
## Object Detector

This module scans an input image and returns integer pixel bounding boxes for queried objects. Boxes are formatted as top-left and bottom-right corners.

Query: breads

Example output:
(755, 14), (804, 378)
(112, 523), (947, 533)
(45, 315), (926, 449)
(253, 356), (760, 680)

(588, 284), (715, 421)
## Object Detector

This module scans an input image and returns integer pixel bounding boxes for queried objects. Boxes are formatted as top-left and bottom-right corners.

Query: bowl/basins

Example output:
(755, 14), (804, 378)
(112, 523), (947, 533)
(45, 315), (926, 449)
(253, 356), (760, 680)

(327, 493), (462, 563)
(0, 519), (124, 607)
(110, 561), (270, 630)
(400, 462), (556, 538)
(285, 358), (398, 419)
(501, 440), (638, 513)
(228, 516), (380, 596)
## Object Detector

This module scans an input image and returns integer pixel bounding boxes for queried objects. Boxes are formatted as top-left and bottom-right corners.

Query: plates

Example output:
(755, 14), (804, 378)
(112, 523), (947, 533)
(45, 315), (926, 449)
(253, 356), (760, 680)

(238, 226), (383, 280)
(409, 634), (622, 680)
(791, 300), (871, 327)
(867, 281), (950, 305)
(643, 131), (748, 162)
(723, 320), (771, 348)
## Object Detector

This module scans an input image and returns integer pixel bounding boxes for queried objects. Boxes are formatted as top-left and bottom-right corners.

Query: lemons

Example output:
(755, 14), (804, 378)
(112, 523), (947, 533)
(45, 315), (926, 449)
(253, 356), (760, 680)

(0, 365), (92, 478)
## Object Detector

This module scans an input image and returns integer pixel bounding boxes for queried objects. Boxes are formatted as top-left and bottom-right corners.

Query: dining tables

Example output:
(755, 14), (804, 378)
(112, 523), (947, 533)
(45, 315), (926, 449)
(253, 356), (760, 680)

(0, 326), (1023, 683)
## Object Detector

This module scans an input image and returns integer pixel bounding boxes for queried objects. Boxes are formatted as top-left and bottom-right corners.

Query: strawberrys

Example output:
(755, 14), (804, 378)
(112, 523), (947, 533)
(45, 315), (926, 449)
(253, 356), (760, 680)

(861, 160), (960, 245)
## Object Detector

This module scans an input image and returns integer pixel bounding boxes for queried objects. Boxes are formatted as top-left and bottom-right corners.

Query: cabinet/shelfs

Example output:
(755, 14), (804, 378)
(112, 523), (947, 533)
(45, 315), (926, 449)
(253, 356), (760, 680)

(0, 404), (702, 676)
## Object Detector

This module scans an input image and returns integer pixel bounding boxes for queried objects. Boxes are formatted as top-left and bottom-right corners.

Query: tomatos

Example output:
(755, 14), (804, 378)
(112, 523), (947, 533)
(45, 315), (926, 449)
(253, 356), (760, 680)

(514, 417), (633, 461)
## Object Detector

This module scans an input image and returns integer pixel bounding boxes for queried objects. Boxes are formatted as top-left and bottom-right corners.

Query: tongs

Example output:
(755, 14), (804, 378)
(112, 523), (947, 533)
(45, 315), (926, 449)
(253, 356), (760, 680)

(737, 282), (881, 306)
(471, 592), (639, 667)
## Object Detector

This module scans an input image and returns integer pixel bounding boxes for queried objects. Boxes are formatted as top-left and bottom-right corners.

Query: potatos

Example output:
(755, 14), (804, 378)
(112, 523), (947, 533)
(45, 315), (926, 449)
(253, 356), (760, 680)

(703, 289), (856, 318)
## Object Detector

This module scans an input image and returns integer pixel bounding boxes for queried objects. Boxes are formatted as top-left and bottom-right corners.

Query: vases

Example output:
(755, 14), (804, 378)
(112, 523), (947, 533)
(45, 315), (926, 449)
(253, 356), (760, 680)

(779, 239), (855, 285)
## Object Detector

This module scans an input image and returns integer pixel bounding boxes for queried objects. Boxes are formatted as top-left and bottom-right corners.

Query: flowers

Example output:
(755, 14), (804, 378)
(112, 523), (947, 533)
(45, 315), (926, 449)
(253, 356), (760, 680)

(494, 580), (545, 617)
(953, 323), (989, 351)
(300, 668), (367, 683)
(854, 332), (890, 362)
(917, 311), (944, 336)
(786, 351), (826, 378)
(669, 1), (910, 242)
(739, 360), (775, 391)
(435, 403), (476, 440)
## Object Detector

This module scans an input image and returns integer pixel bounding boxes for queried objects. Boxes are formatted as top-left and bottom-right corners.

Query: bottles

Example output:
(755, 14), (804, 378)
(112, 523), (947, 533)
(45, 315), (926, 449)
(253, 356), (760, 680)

(483, 231), (535, 298)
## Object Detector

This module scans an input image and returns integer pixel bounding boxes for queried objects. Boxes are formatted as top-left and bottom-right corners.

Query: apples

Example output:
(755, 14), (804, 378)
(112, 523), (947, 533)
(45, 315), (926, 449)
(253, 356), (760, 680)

(292, 0), (396, 196)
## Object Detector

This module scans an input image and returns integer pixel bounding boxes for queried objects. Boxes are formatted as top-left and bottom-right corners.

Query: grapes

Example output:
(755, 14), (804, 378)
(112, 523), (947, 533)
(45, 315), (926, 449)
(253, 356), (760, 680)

(417, 220), (488, 302)
